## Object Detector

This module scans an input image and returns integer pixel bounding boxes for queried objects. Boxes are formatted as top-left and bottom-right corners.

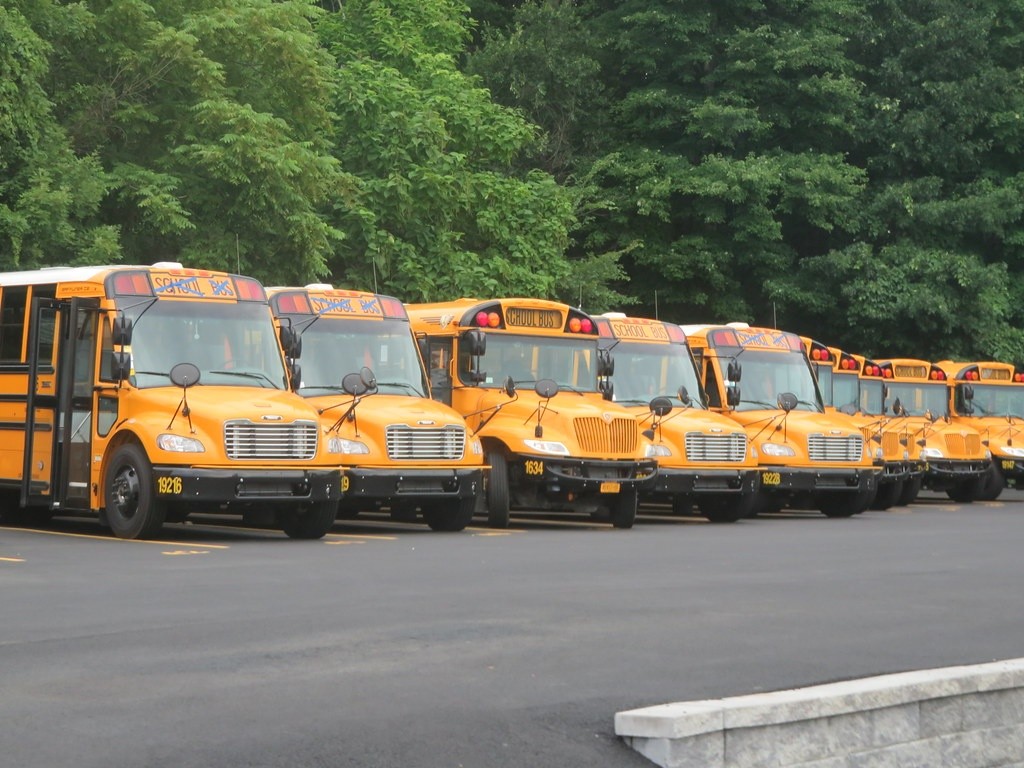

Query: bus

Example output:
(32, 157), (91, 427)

(379, 297), (673, 536)
(531, 307), (1024, 524)
(222, 283), (518, 534)
(0, 260), (378, 541)
